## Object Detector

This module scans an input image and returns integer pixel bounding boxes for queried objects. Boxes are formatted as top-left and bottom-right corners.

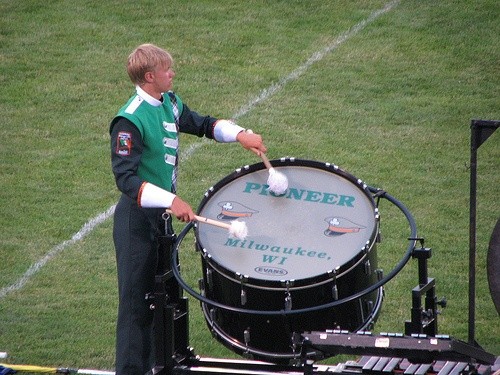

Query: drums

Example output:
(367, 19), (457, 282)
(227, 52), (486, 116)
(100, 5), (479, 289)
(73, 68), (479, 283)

(193, 158), (385, 363)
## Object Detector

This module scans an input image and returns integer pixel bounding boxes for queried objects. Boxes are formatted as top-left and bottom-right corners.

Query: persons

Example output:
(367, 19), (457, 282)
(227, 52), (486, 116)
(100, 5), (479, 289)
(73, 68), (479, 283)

(109, 44), (267, 375)
